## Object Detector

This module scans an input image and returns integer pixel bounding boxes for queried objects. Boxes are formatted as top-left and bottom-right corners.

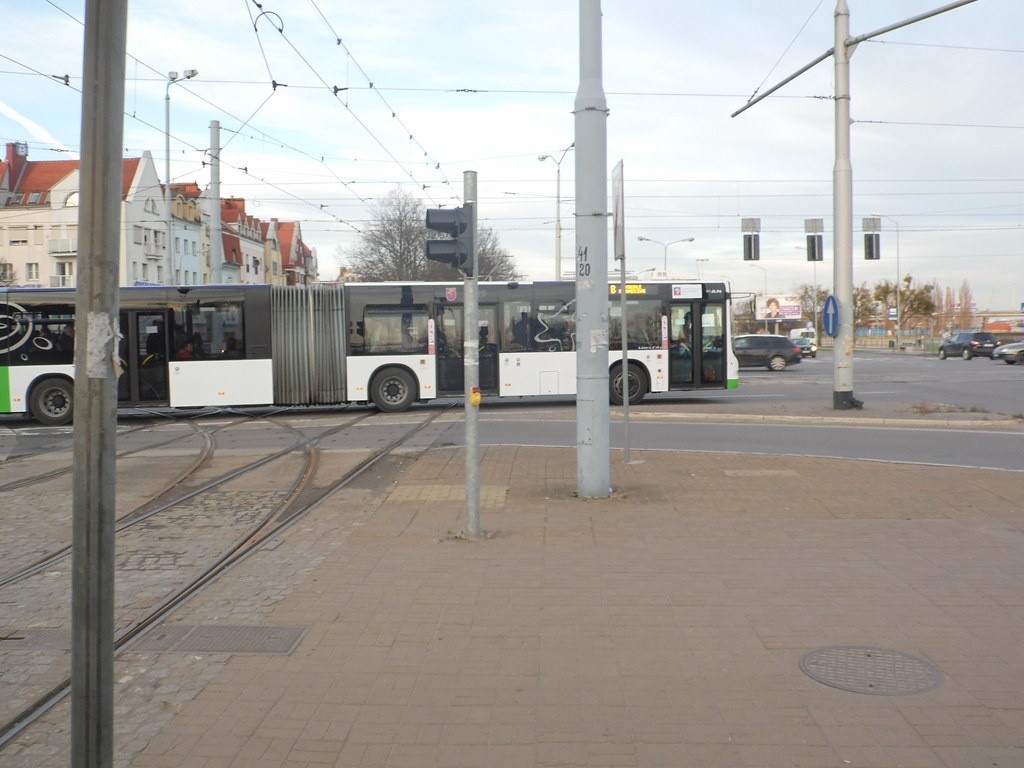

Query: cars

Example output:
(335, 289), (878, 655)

(791, 338), (817, 359)
(992, 338), (1024, 365)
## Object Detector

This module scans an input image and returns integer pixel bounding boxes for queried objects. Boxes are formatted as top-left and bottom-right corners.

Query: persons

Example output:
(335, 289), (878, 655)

(216, 338), (238, 359)
(11, 322), (74, 363)
(437, 330), (449, 389)
(143, 319), (166, 399)
(398, 322), (424, 352)
(766, 298), (785, 318)
(677, 312), (713, 352)
(370, 320), (388, 353)
(634, 318), (654, 343)
(505, 312), (566, 349)
(176, 334), (204, 359)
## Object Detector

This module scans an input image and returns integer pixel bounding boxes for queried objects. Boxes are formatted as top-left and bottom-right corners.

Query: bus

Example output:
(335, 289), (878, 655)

(0, 274), (740, 427)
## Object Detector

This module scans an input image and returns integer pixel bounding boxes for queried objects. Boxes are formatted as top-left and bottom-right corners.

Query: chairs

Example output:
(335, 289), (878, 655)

(192, 333), (208, 358)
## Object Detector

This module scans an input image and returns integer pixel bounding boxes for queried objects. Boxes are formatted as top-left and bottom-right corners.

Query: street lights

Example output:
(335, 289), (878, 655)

(869, 211), (901, 353)
(161, 69), (199, 285)
(637, 235), (694, 272)
(750, 263), (768, 335)
(793, 245), (818, 336)
(537, 141), (575, 279)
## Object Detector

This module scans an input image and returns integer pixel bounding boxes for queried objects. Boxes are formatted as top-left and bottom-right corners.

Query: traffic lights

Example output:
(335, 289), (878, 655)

(424, 201), (475, 277)
(355, 321), (366, 337)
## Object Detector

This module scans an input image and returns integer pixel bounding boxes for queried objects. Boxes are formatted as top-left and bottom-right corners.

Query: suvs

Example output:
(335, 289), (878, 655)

(937, 331), (1002, 361)
(703, 333), (801, 372)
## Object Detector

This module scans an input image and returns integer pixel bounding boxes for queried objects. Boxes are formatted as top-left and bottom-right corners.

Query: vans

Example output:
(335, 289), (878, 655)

(790, 327), (817, 346)
